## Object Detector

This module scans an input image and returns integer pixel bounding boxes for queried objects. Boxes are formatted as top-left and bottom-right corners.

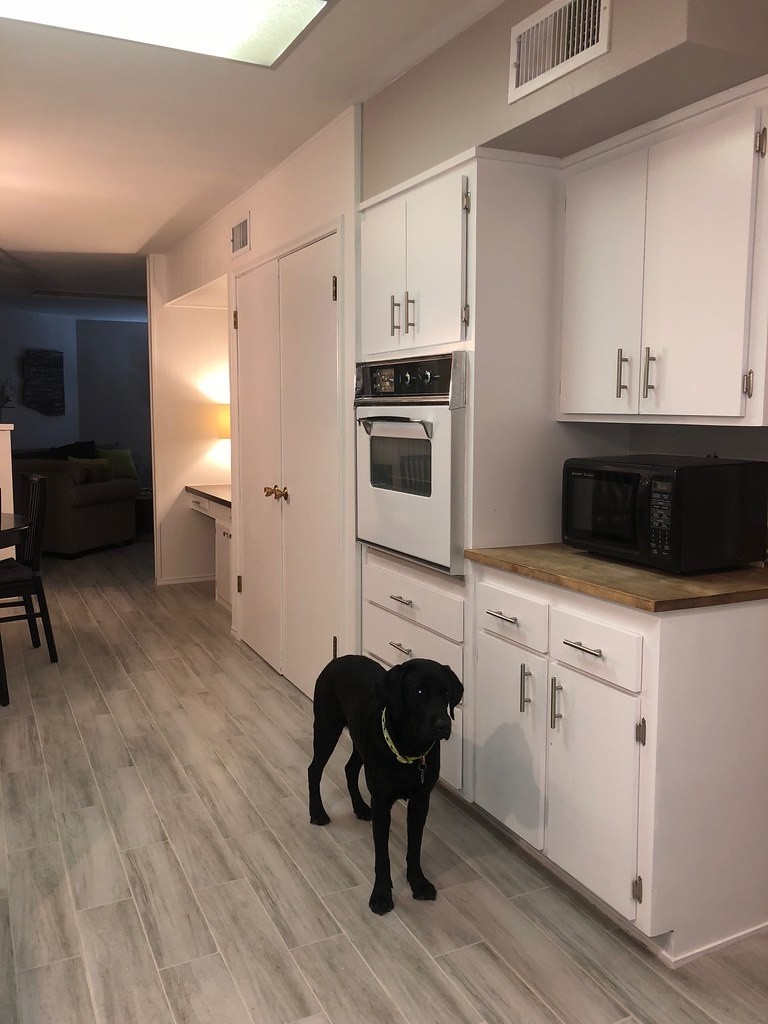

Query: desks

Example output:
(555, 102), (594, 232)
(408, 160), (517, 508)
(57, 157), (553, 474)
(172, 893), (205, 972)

(0, 512), (34, 706)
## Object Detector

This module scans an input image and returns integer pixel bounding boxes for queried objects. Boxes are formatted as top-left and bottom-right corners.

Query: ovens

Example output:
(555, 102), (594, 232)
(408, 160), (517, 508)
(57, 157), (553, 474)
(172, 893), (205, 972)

(354, 351), (466, 577)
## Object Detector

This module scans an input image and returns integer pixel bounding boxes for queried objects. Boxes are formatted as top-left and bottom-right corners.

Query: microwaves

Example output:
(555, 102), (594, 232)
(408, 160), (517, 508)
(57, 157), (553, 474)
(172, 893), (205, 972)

(561, 453), (767, 578)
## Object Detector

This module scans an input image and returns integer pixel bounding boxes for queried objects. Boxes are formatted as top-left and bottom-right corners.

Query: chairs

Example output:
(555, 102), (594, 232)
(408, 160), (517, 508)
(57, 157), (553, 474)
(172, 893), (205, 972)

(0, 471), (59, 664)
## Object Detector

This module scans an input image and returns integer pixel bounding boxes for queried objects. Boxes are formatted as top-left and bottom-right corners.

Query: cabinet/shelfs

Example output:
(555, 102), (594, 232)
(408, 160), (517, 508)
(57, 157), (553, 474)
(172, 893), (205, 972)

(361, 174), (468, 356)
(186, 491), (235, 607)
(559, 105), (763, 417)
(233, 226), (342, 705)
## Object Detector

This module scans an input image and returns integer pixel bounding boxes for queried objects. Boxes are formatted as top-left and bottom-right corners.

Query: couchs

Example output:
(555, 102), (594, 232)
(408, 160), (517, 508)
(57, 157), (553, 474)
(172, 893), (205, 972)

(11, 442), (140, 557)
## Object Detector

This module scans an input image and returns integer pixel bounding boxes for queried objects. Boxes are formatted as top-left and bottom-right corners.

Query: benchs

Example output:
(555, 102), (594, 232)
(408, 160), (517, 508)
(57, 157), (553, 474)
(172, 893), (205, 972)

(472, 581), (644, 922)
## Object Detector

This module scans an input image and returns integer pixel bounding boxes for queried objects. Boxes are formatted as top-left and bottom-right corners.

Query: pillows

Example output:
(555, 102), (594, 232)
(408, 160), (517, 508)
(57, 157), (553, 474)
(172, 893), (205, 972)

(68, 455), (114, 484)
(49, 441), (98, 460)
(92, 447), (138, 480)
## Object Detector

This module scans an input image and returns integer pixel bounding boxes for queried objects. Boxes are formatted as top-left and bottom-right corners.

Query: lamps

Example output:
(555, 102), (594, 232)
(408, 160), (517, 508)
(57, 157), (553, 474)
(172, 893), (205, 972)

(216, 403), (232, 441)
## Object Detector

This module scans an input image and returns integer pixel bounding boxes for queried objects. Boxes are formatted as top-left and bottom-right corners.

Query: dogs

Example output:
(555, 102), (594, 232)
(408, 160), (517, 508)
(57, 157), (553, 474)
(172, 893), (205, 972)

(305, 653), (467, 916)
